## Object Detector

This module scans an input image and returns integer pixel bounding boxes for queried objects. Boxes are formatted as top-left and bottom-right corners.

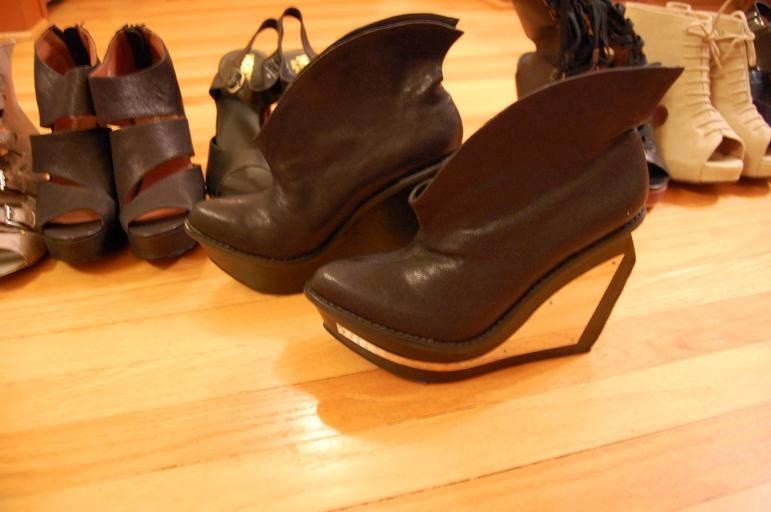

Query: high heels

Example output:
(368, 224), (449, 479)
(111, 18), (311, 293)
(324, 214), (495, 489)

(513, 1), (670, 209)
(184, 11), (466, 294)
(304, 62), (685, 383)
(207, 8), (324, 193)
(667, 1), (770, 180)
(623, 0), (745, 184)
(30, 21), (117, 263)
(89, 24), (207, 261)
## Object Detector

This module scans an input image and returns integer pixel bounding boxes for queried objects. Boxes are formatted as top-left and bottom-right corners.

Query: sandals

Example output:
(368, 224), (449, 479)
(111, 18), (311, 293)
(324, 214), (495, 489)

(0, 41), (46, 279)
